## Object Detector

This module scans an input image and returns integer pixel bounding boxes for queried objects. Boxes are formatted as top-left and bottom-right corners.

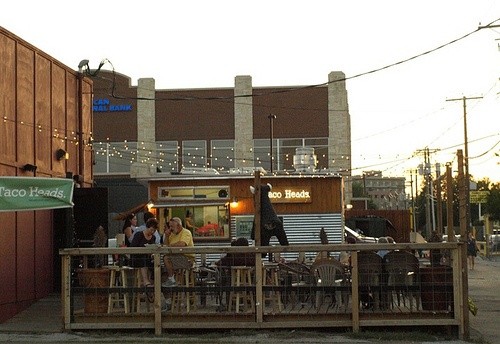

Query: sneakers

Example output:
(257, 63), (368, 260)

(162, 279), (177, 287)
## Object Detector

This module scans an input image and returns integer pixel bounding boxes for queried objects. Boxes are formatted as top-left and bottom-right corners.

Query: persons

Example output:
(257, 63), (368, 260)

(215, 238), (255, 308)
(341, 221), (481, 310)
(123, 210), (193, 247)
(129, 218), (158, 302)
(163, 217), (196, 304)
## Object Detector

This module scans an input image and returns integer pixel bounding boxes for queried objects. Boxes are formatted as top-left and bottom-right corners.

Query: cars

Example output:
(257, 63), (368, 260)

(344, 225), (381, 244)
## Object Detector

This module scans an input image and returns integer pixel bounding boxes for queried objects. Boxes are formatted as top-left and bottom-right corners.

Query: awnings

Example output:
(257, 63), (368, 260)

(151, 201), (229, 208)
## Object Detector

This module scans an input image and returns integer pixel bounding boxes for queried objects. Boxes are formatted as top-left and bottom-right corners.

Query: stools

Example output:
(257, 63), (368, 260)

(107, 262), (278, 313)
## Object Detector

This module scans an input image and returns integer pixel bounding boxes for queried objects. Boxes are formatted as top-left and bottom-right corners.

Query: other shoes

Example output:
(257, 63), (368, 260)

(145, 284), (154, 288)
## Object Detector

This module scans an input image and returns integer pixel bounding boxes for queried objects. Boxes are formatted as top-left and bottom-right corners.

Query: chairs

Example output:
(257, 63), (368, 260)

(310, 251), (420, 313)
